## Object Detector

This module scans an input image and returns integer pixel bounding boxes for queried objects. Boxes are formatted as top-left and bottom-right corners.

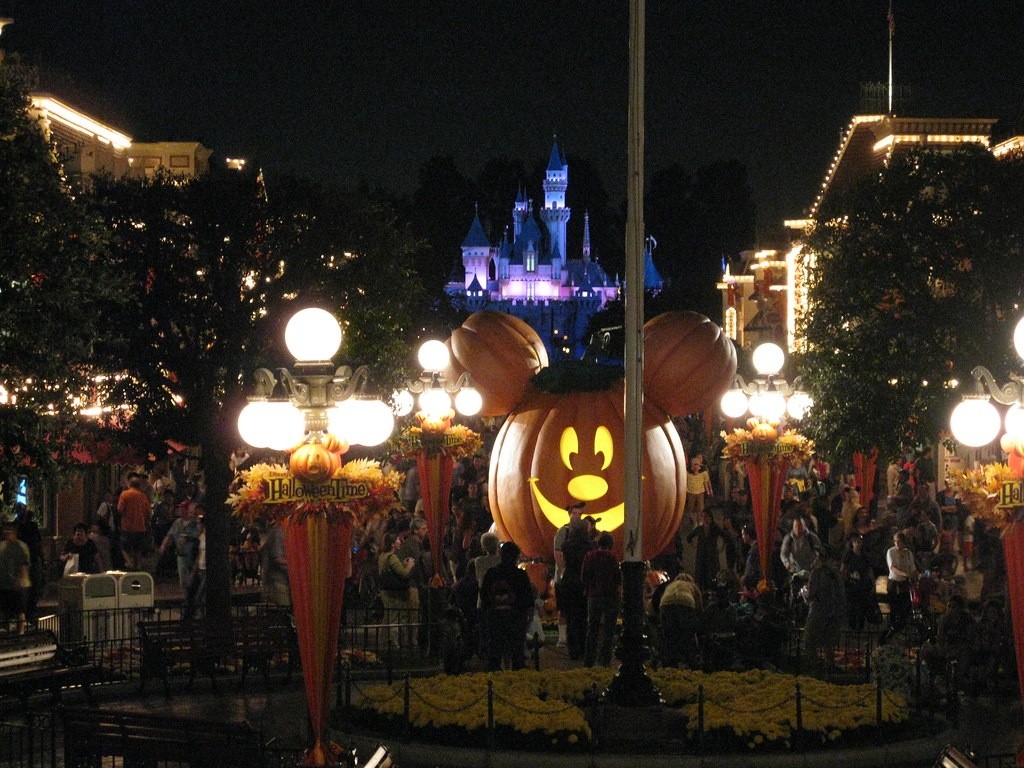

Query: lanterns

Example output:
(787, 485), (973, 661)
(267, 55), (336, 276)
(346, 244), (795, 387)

(441, 310), (737, 592)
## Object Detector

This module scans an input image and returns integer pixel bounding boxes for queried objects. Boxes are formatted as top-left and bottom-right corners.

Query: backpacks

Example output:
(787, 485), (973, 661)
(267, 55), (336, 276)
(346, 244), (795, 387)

(489, 571), (516, 613)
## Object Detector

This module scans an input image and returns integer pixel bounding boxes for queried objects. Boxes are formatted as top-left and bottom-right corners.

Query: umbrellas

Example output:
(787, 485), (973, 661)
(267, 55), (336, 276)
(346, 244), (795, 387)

(1001, 503), (1024, 768)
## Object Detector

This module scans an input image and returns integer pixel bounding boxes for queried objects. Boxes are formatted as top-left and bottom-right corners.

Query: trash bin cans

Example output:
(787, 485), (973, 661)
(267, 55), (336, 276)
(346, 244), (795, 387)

(105, 569), (154, 650)
(57, 572), (119, 651)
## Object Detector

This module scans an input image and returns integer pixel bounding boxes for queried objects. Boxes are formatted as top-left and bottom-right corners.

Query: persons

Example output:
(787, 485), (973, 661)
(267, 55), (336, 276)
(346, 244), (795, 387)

(95, 470), (207, 619)
(779, 448), (1017, 699)
(0, 505), (106, 638)
(227, 519), (294, 669)
(671, 411), (835, 528)
(552, 503), (795, 673)
(339, 415), (537, 675)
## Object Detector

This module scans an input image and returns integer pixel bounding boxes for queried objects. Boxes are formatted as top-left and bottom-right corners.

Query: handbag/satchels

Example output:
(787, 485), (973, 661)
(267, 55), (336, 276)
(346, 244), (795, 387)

(64, 553), (81, 576)
(380, 556), (411, 593)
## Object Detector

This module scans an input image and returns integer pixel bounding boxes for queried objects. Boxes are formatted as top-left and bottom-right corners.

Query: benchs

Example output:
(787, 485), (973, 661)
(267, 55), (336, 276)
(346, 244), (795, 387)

(0, 629), (123, 726)
(364, 741), (399, 768)
(60, 706), (297, 768)
(137, 617), (299, 701)
(933, 744), (978, 768)
